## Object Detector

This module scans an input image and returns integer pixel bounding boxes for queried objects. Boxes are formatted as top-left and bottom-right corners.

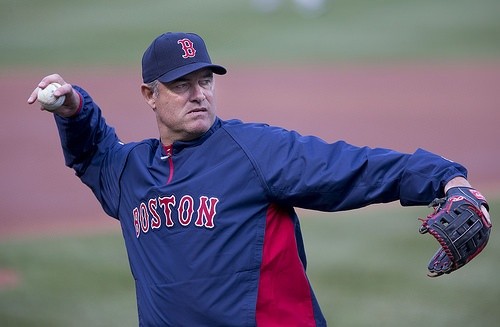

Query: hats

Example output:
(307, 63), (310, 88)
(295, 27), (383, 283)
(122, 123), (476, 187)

(142, 33), (227, 83)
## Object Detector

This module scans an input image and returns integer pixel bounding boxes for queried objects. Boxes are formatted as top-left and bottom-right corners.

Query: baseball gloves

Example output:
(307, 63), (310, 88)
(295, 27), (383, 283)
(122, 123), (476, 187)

(417, 186), (492, 278)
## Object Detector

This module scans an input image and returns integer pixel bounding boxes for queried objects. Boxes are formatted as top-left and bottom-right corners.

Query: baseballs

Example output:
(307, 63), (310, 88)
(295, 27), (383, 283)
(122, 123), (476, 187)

(37, 83), (66, 111)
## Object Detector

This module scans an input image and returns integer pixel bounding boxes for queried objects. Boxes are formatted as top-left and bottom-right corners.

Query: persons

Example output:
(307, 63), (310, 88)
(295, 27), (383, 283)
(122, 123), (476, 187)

(27, 30), (492, 327)
